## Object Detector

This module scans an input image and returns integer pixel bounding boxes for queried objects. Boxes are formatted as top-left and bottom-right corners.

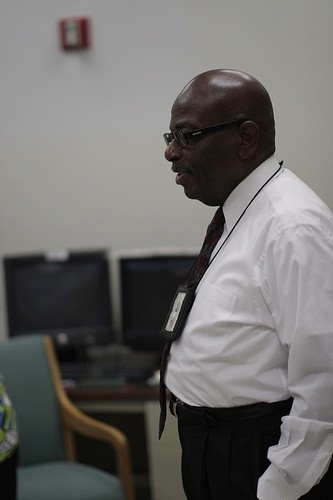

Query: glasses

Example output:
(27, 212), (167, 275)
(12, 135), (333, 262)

(163, 119), (244, 148)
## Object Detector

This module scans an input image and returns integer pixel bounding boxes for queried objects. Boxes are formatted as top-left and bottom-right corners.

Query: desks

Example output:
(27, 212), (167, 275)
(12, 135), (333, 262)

(65, 358), (172, 401)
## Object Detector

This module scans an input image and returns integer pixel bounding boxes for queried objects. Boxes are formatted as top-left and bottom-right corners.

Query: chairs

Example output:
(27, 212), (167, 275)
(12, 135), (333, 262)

(0, 335), (133, 500)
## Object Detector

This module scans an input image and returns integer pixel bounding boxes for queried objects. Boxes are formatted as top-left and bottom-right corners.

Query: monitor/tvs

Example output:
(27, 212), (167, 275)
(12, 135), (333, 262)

(120, 255), (198, 351)
(3, 252), (116, 344)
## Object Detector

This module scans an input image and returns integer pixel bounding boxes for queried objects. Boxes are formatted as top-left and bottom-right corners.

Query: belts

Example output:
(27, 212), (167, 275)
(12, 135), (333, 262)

(169, 394), (292, 426)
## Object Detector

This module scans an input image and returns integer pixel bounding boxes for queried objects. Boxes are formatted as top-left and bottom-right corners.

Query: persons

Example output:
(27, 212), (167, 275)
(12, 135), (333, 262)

(165, 69), (333, 500)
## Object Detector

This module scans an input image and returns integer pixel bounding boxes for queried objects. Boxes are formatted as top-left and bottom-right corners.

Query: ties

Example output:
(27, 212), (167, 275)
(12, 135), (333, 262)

(159, 207), (225, 440)
